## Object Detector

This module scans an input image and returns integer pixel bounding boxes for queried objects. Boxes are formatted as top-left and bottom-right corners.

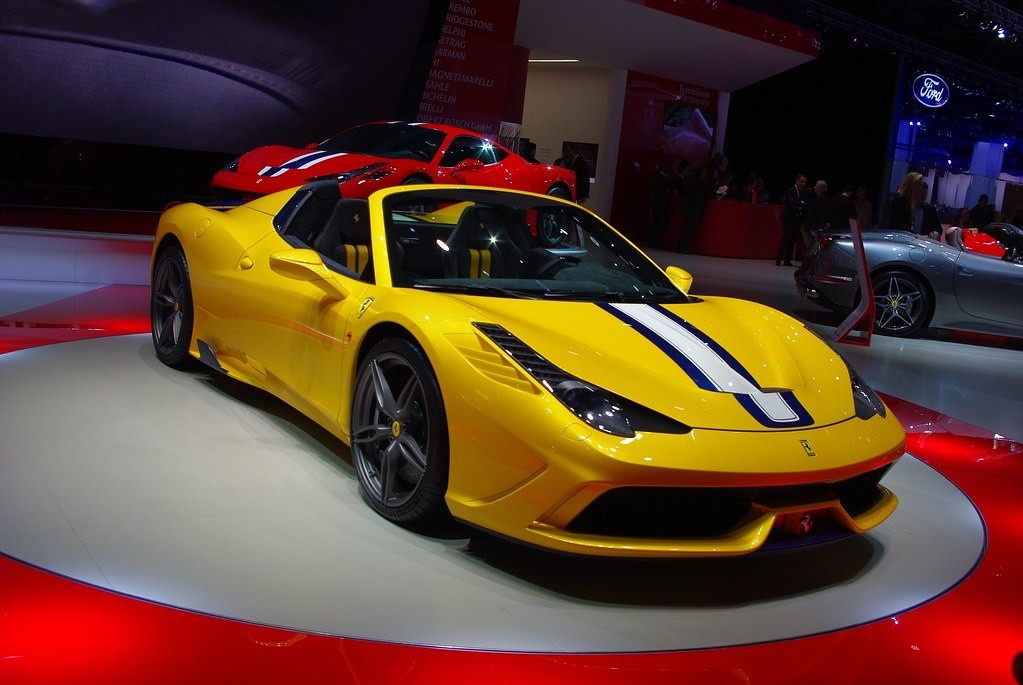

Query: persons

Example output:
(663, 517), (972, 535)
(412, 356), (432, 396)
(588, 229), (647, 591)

(989, 203), (995, 210)
(563, 143), (590, 202)
(775, 173), (806, 266)
(971, 193), (993, 227)
(553, 155), (569, 168)
(798, 187), (814, 245)
(739, 169), (766, 203)
(993, 212), (1005, 223)
(938, 204), (959, 217)
(676, 165), (709, 255)
(673, 161), (690, 192)
(881, 171), (924, 231)
(650, 165), (672, 248)
(951, 207), (975, 227)
(520, 142), (540, 163)
(832, 183), (856, 234)
(912, 181), (942, 236)
(852, 184), (874, 230)
(808, 180), (829, 230)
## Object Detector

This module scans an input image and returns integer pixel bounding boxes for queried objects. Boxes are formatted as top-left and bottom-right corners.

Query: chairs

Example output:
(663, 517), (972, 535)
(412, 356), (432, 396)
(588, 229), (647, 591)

(312, 198), (414, 287)
(945, 227), (970, 253)
(442, 202), (527, 278)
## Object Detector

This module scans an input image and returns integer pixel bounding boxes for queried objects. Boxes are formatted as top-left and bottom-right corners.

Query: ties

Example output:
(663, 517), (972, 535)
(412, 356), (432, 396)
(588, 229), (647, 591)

(914, 205), (922, 234)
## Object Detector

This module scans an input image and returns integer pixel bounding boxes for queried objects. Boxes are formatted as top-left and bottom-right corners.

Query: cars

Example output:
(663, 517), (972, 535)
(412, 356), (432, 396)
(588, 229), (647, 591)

(793, 221), (1023, 347)
(215, 118), (579, 253)
(141, 180), (907, 572)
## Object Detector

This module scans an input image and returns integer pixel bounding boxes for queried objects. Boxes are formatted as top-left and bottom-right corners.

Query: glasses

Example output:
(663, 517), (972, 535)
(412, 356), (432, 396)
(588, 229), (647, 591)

(799, 180), (806, 184)
